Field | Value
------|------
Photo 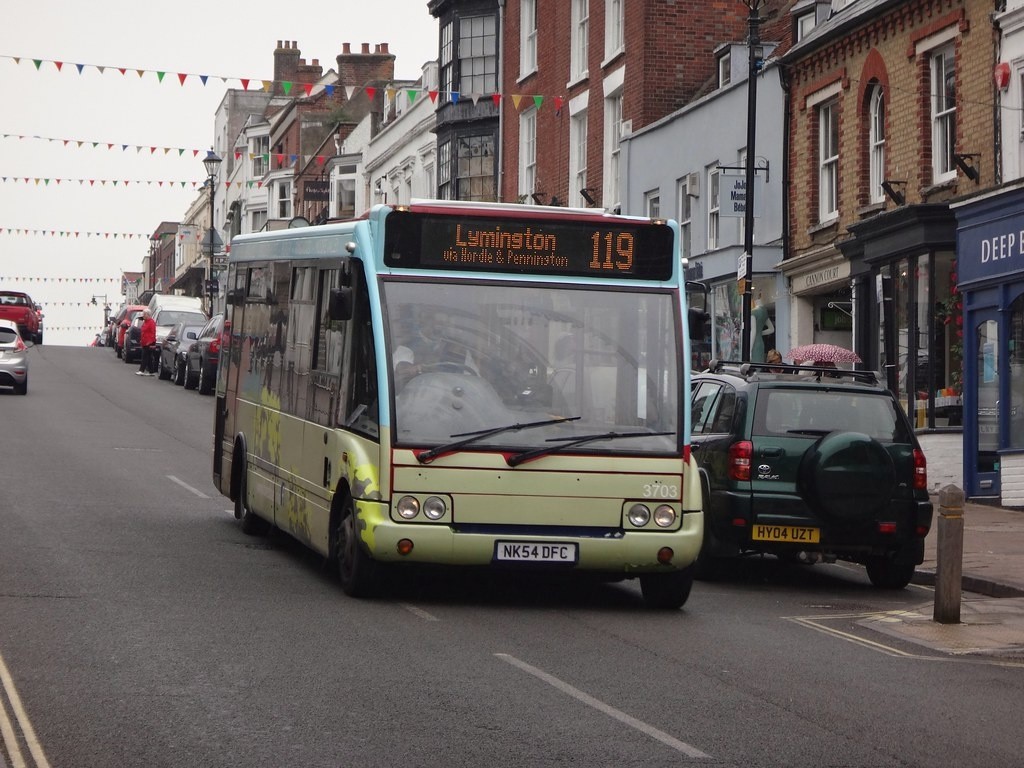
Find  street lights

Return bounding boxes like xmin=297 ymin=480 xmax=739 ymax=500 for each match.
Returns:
xmin=202 ymin=149 xmax=223 ymax=322
xmin=90 ymin=293 xmax=107 ymax=327
xmin=149 ymin=231 xmax=162 ymax=295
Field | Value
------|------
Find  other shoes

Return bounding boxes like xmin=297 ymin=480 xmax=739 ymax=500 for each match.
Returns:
xmin=135 ymin=371 xmax=144 ymax=376
xmin=145 ymin=372 xmax=155 ymax=376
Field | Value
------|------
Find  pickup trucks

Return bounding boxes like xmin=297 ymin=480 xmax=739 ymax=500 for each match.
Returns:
xmin=0 ymin=290 xmax=45 ymax=345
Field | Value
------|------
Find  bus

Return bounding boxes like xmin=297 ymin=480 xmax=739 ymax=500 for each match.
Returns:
xmin=207 ymin=197 xmax=717 ymax=613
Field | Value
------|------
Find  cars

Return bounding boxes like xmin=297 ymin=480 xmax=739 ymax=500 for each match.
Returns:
xmin=98 ymin=292 xmax=231 ymax=395
xmin=0 ymin=317 xmax=35 ymax=395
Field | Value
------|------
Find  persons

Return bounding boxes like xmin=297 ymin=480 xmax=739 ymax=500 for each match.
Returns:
xmin=748 ymin=299 xmax=775 ymax=363
xmin=135 ymin=308 xmax=157 ymax=377
xmin=763 ymin=348 xmax=791 ymax=373
xmin=392 ymin=298 xmax=481 ymax=383
xmin=811 ymin=360 xmax=841 ymax=378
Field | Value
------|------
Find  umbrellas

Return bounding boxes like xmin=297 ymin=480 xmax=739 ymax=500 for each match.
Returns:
xmin=786 ymin=344 xmax=863 ymax=363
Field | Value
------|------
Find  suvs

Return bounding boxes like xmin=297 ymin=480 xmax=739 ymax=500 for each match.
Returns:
xmin=683 ymin=357 xmax=935 ymax=592
